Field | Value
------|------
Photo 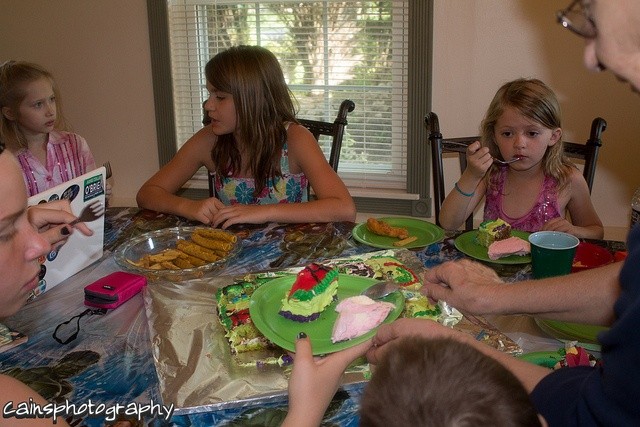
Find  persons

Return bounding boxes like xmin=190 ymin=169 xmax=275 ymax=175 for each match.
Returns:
xmin=0 ymin=138 xmax=373 ymax=427
xmin=366 ymin=0 xmax=637 ymax=427
xmin=137 ymin=44 xmax=356 ymax=230
xmin=437 ymin=77 xmax=604 ymax=241
xmin=357 ymin=333 xmax=550 ymax=427
xmin=0 ymin=61 xmax=113 ymax=200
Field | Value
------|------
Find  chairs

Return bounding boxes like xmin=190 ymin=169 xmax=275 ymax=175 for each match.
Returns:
xmin=421 ymin=110 xmax=608 ymax=234
xmin=199 ymin=98 xmax=356 ymax=202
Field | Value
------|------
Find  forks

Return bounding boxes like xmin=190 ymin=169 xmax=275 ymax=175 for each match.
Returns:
xmin=441 ymin=141 xmax=520 ymax=166
xmin=102 ymin=161 xmax=113 ymax=180
xmin=69 ymin=200 xmax=107 ymax=227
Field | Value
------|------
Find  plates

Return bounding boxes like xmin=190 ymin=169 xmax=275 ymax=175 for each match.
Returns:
xmin=249 ymin=273 xmax=405 ymax=356
xmin=571 ymin=242 xmax=614 ymax=273
xmin=351 ymin=217 xmax=443 ymax=249
xmin=113 ymin=224 xmax=243 ymax=281
xmin=454 ymin=230 xmax=533 ymax=264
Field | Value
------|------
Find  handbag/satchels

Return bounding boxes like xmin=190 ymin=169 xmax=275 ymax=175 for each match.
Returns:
xmin=51 ymin=270 xmax=148 ymax=345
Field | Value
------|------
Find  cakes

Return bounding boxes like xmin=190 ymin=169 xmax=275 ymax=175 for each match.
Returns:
xmin=549 ymin=340 xmax=597 ymax=374
xmin=216 ymin=254 xmax=443 ymax=381
xmin=476 ymin=218 xmax=512 ymax=248
xmin=279 ymin=262 xmax=339 ymax=320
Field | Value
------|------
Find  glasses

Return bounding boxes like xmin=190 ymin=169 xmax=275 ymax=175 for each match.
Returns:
xmin=557 ymin=2 xmax=599 ymax=39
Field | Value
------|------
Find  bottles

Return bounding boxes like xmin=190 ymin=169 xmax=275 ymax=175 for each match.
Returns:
xmin=630 ymin=188 xmax=640 ymax=233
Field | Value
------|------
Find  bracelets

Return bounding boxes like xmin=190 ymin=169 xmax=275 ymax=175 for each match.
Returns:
xmin=453 ymin=182 xmax=474 ymax=197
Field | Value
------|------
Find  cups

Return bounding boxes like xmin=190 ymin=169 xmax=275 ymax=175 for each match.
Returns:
xmin=528 ymin=230 xmax=580 ymax=279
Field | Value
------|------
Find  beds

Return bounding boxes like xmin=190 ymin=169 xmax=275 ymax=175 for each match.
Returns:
xmin=0 ymin=207 xmax=640 ymax=426
